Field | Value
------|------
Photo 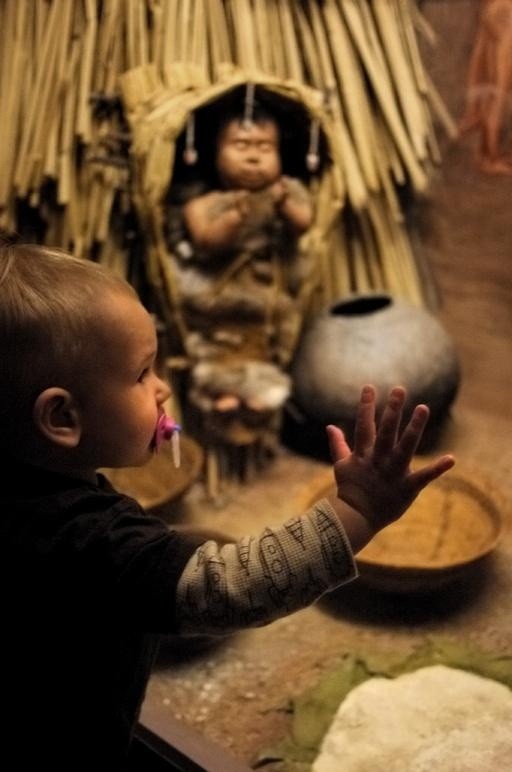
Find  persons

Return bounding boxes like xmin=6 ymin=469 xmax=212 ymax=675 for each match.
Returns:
xmin=179 ymin=98 xmax=316 ymax=415
xmin=0 ymin=248 xmax=454 ymax=772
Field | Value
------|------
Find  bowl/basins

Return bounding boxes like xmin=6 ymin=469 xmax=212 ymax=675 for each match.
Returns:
xmin=305 ymin=465 xmax=507 ymax=594
xmin=95 ymin=435 xmax=204 ymax=523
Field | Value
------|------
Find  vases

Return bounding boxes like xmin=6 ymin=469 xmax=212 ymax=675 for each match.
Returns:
xmin=285 ymin=290 xmax=461 ymax=458
